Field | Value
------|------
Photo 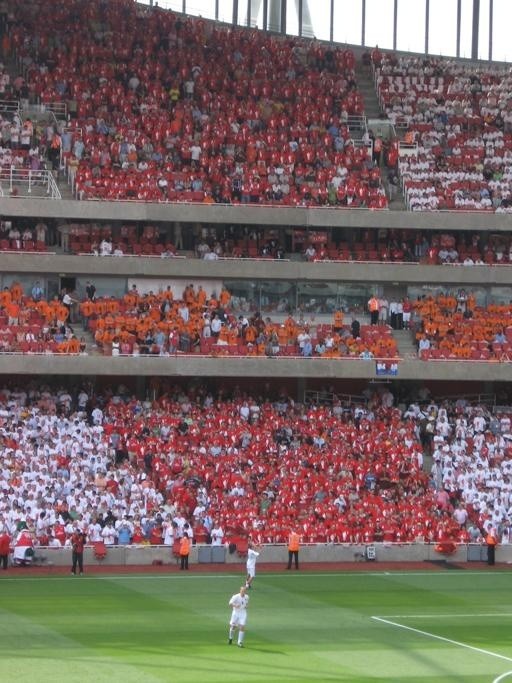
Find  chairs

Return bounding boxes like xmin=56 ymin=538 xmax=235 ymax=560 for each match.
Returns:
xmin=0 ymin=0 xmax=511 ymax=362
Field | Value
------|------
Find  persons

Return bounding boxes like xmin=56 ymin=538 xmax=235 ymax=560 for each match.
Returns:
xmin=2 ymin=218 xmax=512 ymax=265
xmin=227 ymin=586 xmax=250 ymax=647
xmin=71 ymin=529 xmax=86 ymax=573
xmin=486 ymin=523 xmax=498 ymax=565
xmin=0 ymin=531 xmax=11 ymax=568
xmin=1 ymin=0 xmax=511 ymax=213
xmin=244 ymin=542 xmax=266 ymax=589
xmin=0 ymin=379 xmax=512 ymax=545
xmin=0 ymin=280 xmax=512 ymax=362
xmin=179 ymin=532 xmax=192 ymax=569
xmin=287 ymin=528 xmax=300 ymax=569
